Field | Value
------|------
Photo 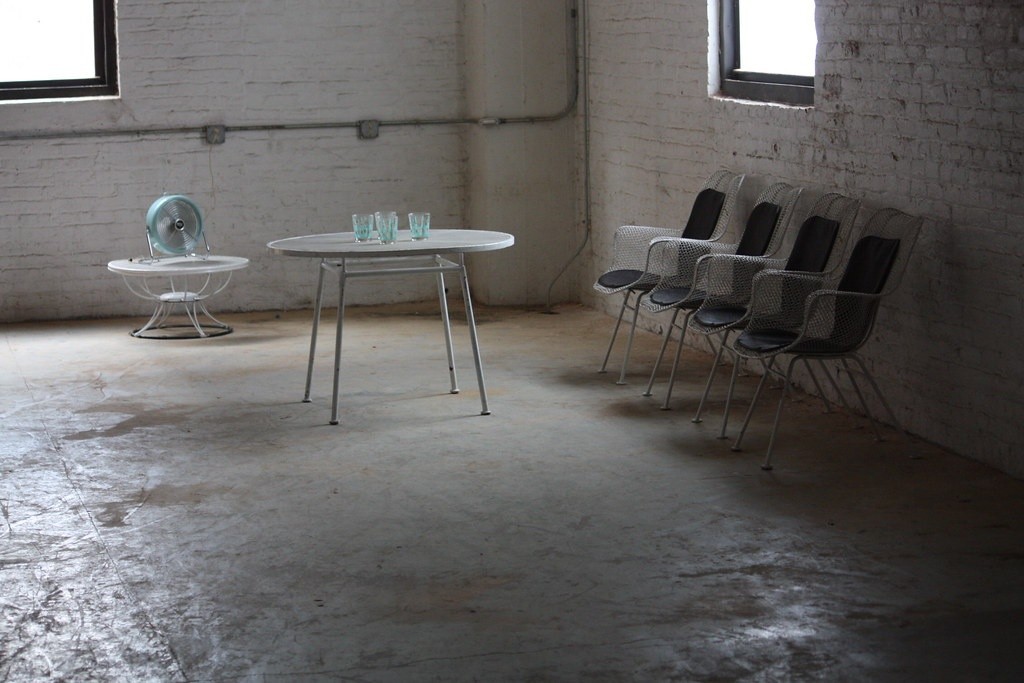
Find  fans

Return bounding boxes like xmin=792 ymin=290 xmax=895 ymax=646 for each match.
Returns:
xmin=138 ymin=194 xmax=211 ymax=266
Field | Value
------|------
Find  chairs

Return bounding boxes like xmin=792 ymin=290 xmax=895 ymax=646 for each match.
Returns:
xmin=593 ymin=171 xmax=927 ymax=469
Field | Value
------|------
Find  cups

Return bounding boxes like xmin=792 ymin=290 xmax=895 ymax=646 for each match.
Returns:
xmin=375 ymin=211 xmax=398 ymax=245
xmin=352 ymin=213 xmax=374 ymax=243
xmin=407 ymin=212 xmax=430 ymax=242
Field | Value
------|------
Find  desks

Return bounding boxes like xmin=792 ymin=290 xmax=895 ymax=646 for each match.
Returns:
xmin=107 ymin=253 xmax=249 ymax=339
xmin=267 ymin=227 xmax=514 ymax=425
